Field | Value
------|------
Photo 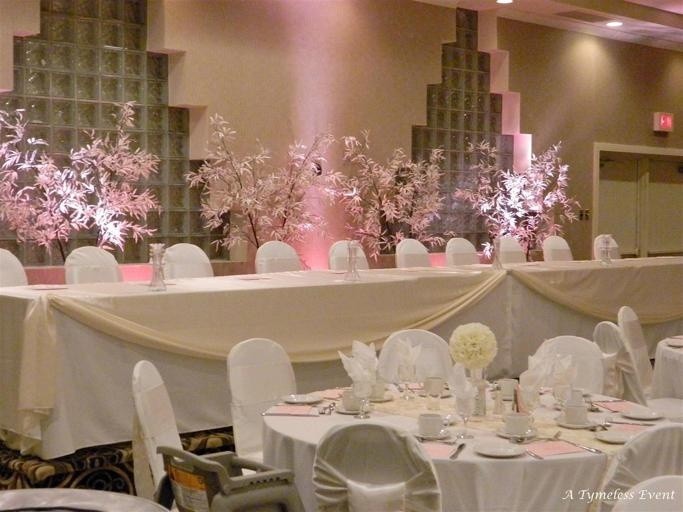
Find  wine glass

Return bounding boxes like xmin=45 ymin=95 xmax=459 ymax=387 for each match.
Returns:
xmin=455 ymin=396 xmax=473 ymax=439
xmin=352 ymin=380 xmax=371 ymax=420
xmin=394 ymin=358 xmax=417 ymax=401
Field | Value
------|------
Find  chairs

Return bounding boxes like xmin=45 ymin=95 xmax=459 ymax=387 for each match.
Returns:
xmin=528 ymin=333 xmax=605 ymax=396
xmin=160 ymin=242 xmax=215 ymax=278
xmin=131 ymin=359 xmax=260 ymax=508
xmin=445 ymin=237 xmax=479 ymax=263
xmin=225 ymin=337 xmax=300 ymax=477
xmin=498 ymin=237 xmax=526 ymax=263
xmin=541 ymin=236 xmax=574 ymax=261
xmin=255 ymin=240 xmax=303 ymax=274
xmin=154 ymin=441 xmax=306 ymax=510
xmin=311 ymin=417 xmax=443 ymax=511
xmin=591 ymin=420 xmax=682 ymax=512
xmin=63 ymin=245 xmax=125 ymax=283
xmin=328 ymin=240 xmax=368 ymax=269
xmin=0 ymin=248 xmax=29 ymax=286
xmin=593 ymin=234 xmax=622 ymax=258
xmin=614 ymin=472 xmax=680 ymax=510
xmin=395 ymin=238 xmax=431 ymax=268
xmin=378 ymin=327 xmax=452 ymax=380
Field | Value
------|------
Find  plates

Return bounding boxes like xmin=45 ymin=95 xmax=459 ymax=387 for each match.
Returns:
xmin=667 ymin=339 xmax=682 ymax=347
xmin=336 ymin=406 xmax=370 ymax=414
xmin=420 ymin=391 xmax=450 ymax=398
xmin=279 ymin=393 xmax=324 ymax=405
xmin=414 ymin=428 xmax=451 ymax=440
xmin=371 ymin=394 xmax=393 ymax=402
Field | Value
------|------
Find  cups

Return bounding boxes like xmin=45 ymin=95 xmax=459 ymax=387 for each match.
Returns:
xmin=417 ymin=412 xmax=440 ymax=435
xmin=341 ymin=390 xmax=361 ymax=411
xmin=418 ymin=376 xmax=444 ymax=395
xmin=370 ymin=380 xmax=383 ymax=400
xmin=473 ymin=376 xmax=666 ymax=456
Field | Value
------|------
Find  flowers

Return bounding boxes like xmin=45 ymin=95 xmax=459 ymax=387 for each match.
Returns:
xmin=0 ymin=89 xmax=164 ymax=259
xmin=186 ymin=108 xmax=329 ymax=253
xmin=316 ymin=120 xmax=443 ymax=261
xmin=453 ymin=130 xmax=579 ymax=257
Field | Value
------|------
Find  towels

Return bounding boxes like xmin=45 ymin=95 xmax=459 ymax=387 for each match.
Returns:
xmin=339 ymin=338 xmax=379 ymax=390
xmin=528 ymin=344 xmax=552 ymax=378
xmin=549 ymin=351 xmax=578 ymax=384
xmin=453 ymin=362 xmax=478 ymax=417
xmin=393 ymin=333 xmax=421 ymax=367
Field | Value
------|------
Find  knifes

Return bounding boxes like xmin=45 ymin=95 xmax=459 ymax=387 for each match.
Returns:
xmin=145 ymin=283 xmax=176 ymax=285
xmin=33 ymin=287 xmax=67 ymax=291
xmin=393 ymin=382 xmax=403 ymax=392
xmin=240 ymin=277 xmax=268 ymax=281
xmin=322 ymin=396 xmax=339 ymax=401
xmin=449 ymin=444 xmax=467 ymax=465
xmin=261 ymin=411 xmax=320 ymax=417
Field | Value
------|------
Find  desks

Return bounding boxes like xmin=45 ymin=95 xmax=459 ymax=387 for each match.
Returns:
xmin=1 ymin=247 xmax=508 ymax=460
xmin=502 ymin=253 xmax=681 ymax=383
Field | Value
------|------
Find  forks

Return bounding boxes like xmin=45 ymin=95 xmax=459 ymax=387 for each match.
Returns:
xmin=319 ymin=401 xmax=335 ymax=415
